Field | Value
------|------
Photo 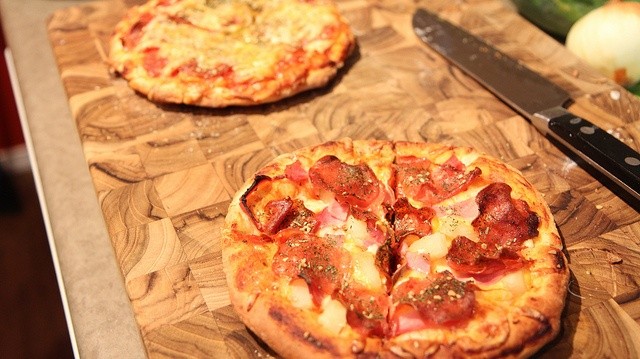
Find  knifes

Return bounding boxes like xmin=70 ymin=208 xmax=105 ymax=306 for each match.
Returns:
xmin=412 ymin=8 xmax=640 ymax=207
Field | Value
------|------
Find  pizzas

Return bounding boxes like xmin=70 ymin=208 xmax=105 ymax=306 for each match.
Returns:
xmin=220 ymin=137 xmax=571 ymax=359
xmin=107 ymin=0 xmax=356 ymax=108
